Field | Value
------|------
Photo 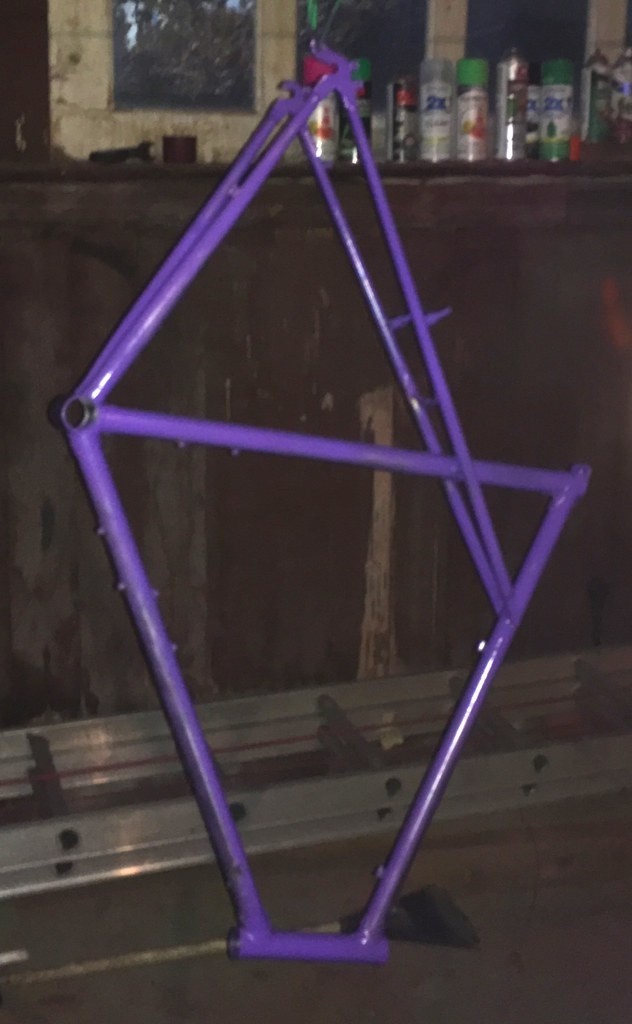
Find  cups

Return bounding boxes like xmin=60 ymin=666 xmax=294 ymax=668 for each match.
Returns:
xmin=162 ymin=136 xmax=196 ymax=163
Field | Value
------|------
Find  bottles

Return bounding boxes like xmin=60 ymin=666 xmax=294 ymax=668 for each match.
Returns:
xmin=304 ymin=45 xmax=632 ymax=173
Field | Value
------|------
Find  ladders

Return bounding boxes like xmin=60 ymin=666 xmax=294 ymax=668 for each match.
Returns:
xmin=0 ymin=641 xmax=630 ymax=903
